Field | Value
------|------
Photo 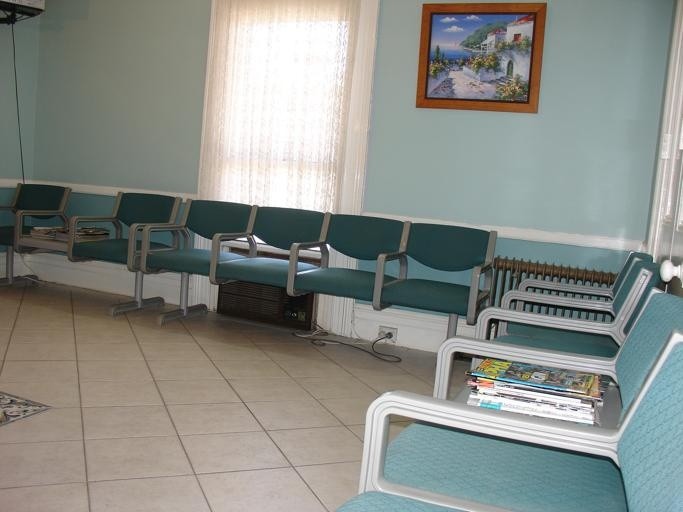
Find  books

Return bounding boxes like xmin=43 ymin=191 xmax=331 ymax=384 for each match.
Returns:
xmin=463 ymin=357 xmax=604 ymax=426
xmin=28 ymin=224 xmax=110 ymax=243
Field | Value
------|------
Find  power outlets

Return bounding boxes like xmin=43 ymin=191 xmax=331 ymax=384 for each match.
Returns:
xmin=377 ymin=325 xmax=398 ymax=344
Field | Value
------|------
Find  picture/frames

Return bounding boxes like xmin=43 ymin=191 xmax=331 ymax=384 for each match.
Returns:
xmin=414 ymin=2 xmax=548 ymax=114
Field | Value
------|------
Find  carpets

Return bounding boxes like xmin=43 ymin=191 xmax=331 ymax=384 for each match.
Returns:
xmin=0 ymin=389 xmax=53 ymax=430
